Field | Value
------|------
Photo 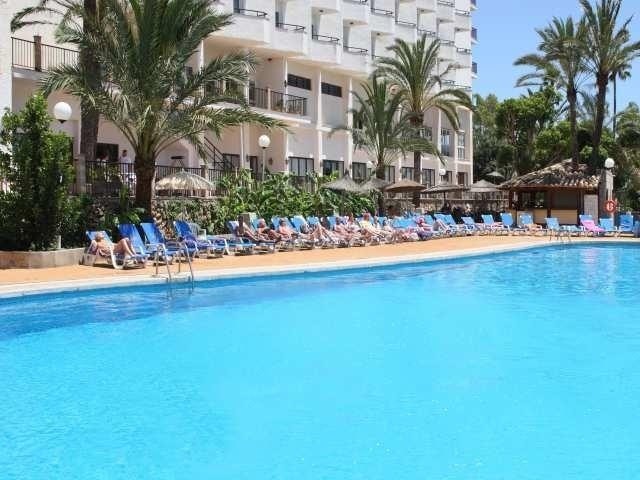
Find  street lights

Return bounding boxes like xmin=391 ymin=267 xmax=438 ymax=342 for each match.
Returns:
xmin=54 ymin=102 xmax=72 ymax=131
xmin=258 ymin=135 xmax=270 ymax=192
xmin=604 ymin=158 xmax=614 ymax=209
xmin=439 ymin=169 xmax=445 ymax=181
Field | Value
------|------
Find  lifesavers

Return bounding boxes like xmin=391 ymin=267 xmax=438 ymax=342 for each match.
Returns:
xmin=601 ymin=199 xmax=616 ymax=212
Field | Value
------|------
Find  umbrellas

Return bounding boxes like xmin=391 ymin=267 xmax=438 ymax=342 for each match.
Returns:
xmin=485 ymin=169 xmax=507 ymax=186
xmin=465 ymin=177 xmax=503 ymax=202
xmin=357 ymin=175 xmax=392 ymax=201
xmin=155 ymin=168 xmax=217 ymax=214
xmin=419 ymin=181 xmax=470 ymax=202
xmin=319 ymin=169 xmax=363 ymax=214
xmin=382 ymin=176 xmax=427 ymax=210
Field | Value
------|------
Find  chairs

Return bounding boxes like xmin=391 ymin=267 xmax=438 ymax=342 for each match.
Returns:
xmin=225 ymin=211 xmax=472 ymax=254
xmin=83 ymin=216 xmax=230 ymax=268
xmin=461 ymin=212 xmax=640 ymax=240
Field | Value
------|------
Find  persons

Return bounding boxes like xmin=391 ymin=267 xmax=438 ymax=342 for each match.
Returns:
xmin=118 ymin=149 xmax=132 ymax=182
xmin=94 ymin=232 xmax=135 ymax=259
xmin=278 ymin=218 xmax=315 ymax=242
xmin=333 ymin=201 xmax=452 ymax=247
xmin=95 ymin=150 xmax=109 ymax=175
xmin=256 ymin=218 xmax=285 ymax=243
xmin=235 ymin=215 xmax=264 ymax=244
xmin=300 ymin=221 xmax=335 ymax=243
